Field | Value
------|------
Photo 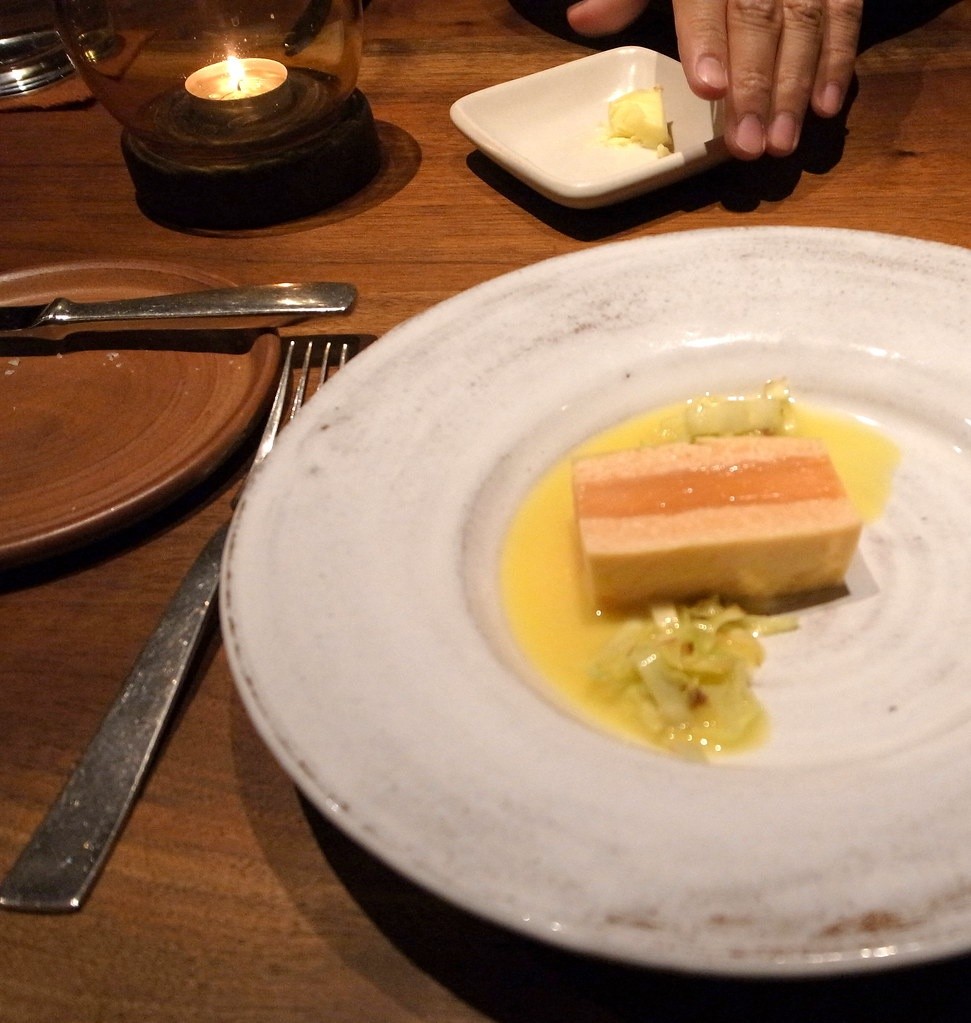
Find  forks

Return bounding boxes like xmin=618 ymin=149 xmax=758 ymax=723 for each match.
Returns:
xmin=0 ymin=341 xmax=348 ymax=913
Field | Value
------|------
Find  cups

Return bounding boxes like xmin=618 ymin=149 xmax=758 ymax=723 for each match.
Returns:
xmin=0 ymin=0 xmax=115 ymax=97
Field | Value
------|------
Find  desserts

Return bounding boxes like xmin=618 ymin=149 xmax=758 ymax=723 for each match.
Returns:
xmin=570 ymin=435 xmax=862 ymax=602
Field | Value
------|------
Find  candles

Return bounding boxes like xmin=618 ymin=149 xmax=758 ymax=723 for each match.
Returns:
xmin=184 ymin=58 xmax=288 ymax=124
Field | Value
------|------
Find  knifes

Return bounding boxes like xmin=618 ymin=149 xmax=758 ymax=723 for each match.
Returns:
xmin=0 ymin=283 xmax=358 ymax=330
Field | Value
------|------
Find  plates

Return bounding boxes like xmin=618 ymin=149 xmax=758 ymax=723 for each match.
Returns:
xmin=0 ymin=259 xmax=280 ymax=553
xmin=220 ymin=226 xmax=970 ymax=977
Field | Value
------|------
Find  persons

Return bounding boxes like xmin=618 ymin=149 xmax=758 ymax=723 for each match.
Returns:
xmin=566 ymin=0 xmax=864 ymax=159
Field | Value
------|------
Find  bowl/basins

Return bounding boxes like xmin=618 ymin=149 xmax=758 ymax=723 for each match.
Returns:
xmin=451 ymin=46 xmax=731 ymax=208
